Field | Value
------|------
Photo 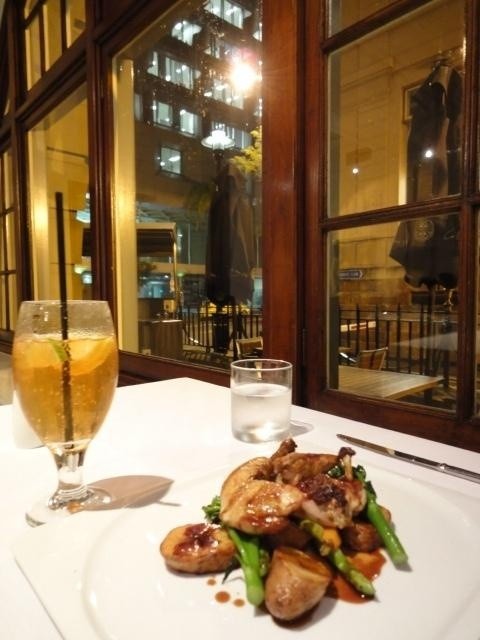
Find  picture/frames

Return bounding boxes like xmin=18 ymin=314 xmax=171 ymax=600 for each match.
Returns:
xmin=401 ymin=76 xmax=426 ymax=125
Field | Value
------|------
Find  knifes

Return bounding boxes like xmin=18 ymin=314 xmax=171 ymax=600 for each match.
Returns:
xmin=338 ymin=430 xmax=479 ymax=483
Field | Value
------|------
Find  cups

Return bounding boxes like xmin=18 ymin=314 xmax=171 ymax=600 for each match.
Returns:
xmin=228 ymin=359 xmax=294 ymax=441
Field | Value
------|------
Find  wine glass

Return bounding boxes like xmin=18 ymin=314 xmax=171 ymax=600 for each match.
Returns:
xmin=12 ymin=295 xmax=117 ymax=526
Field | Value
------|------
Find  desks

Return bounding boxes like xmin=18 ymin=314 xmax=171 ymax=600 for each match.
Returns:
xmin=337 ymin=364 xmax=446 ymax=405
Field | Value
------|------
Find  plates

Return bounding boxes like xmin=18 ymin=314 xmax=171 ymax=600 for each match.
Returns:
xmin=14 ymin=443 xmax=478 ymax=638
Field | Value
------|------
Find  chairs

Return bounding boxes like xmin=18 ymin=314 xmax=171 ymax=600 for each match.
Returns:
xmin=235 ymin=335 xmax=263 ymax=362
xmin=355 ymin=346 xmax=389 ymax=370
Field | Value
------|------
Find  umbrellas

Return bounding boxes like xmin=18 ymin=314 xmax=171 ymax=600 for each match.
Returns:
xmin=203 ymin=159 xmax=256 ymax=341
xmin=388 ymin=57 xmax=464 ymax=402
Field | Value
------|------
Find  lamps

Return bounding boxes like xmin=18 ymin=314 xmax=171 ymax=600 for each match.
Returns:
xmin=201 ymin=1 xmax=237 ymax=161
